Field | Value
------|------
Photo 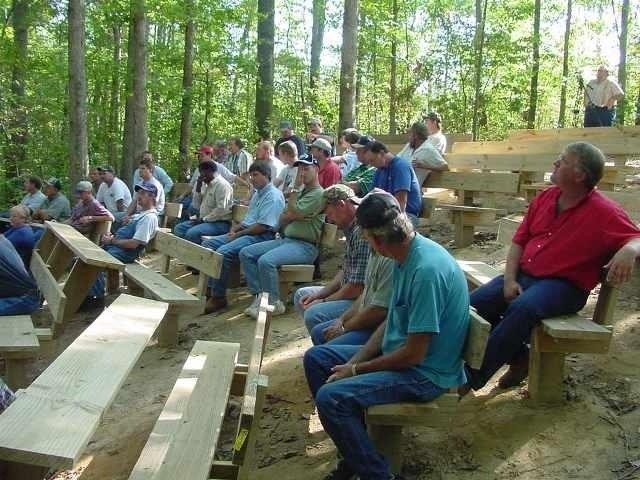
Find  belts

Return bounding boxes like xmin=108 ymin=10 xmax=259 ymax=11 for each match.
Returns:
xmin=286 ymin=236 xmax=316 ymax=247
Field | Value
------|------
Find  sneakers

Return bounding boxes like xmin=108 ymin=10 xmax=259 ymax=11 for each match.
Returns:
xmin=82 ymin=295 xmax=105 ymax=307
xmin=249 ymin=301 xmax=286 ymax=320
xmin=205 ymin=296 xmax=227 ymax=314
xmin=464 ymin=364 xmax=488 ymax=391
xmin=321 ymin=460 xmax=355 ymax=480
xmin=243 ymin=292 xmax=262 ymax=316
xmin=498 ymin=351 xmax=529 ymax=389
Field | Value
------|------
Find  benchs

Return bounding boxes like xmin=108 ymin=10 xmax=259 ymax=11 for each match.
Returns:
xmin=368 ymin=306 xmax=494 ymax=478
xmin=93 ymin=129 xmax=639 ymax=346
xmin=455 ymin=214 xmax=626 ymax=408
xmin=123 ymin=289 xmax=278 ymax=479
xmin=1 ymin=249 xmax=67 ymax=362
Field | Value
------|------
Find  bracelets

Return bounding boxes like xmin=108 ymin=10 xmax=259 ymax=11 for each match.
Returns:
xmin=351 ymin=363 xmax=358 ymax=376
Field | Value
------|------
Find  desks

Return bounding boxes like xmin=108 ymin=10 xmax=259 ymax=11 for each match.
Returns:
xmin=41 ymin=219 xmax=127 ymax=288
xmin=0 ymin=293 xmax=175 ymax=479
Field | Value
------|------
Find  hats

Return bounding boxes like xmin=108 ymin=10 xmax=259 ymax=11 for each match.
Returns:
xmin=421 ymin=112 xmax=441 ymax=123
xmin=96 ymin=164 xmax=114 ymax=172
xmin=406 ymin=123 xmax=428 ymax=141
xmin=355 ymin=192 xmax=402 ymax=228
xmin=193 ymin=145 xmax=214 ymax=154
xmin=322 ymin=184 xmax=365 ymax=206
xmin=135 ymin=183 xmax=157 ymax=194
xmin=43 ymin=178 xmax=61 ymax=189
xmin=293 ymin=135 xmax=376 ymax=167
xmin=72 ymin=181 xmax=93 ymax=193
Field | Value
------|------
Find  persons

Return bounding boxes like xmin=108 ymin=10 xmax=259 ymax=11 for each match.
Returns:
xmin=0 ymin=379 xmax=15 ymax=409
xmin=174 ymin=112 xmax=448 ymax=319
xmin=583 ymin=65 xmax=625 ymax=127
xmin=300 ymin=192 xmax=471 ymax=480
xmin=458 ymin=141 xmax=640 ymax=397
xmin=303 ymin=187 xmax=413 ymax=456
xmin=0 ymin=152 xmax=173 ymax=315
